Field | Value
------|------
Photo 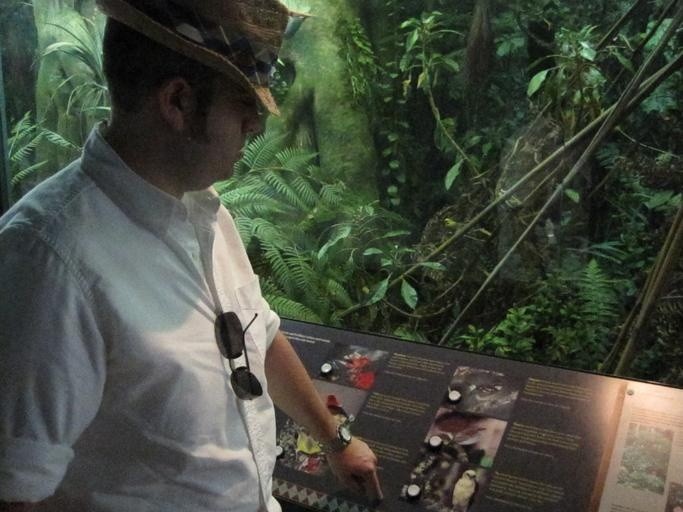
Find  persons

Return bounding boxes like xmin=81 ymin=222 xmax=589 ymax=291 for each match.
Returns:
xmin=0 ymin=0 xmax=384 ymax=512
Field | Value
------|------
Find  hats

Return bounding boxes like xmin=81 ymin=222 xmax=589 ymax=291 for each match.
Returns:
xmin=98 ymin=0 xmax=288 ymax=119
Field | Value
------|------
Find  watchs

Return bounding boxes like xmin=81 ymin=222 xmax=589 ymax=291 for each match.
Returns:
xmin=319 ymin=425 xmax=352 ymax=453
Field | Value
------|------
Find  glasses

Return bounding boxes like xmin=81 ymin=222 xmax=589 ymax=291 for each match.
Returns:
xmin=214 ymin=309 xmax=262 ymax=401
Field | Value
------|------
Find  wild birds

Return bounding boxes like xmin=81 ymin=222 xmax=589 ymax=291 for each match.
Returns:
xmin=451 ymin=470 xmax=476 ymax=512
